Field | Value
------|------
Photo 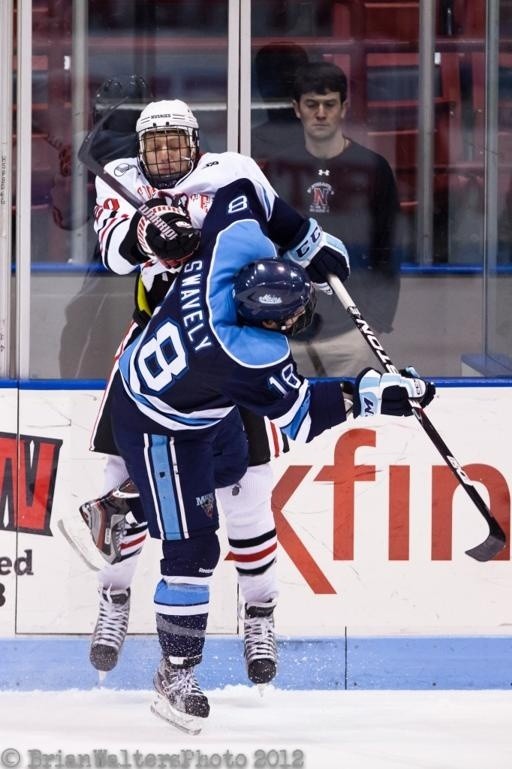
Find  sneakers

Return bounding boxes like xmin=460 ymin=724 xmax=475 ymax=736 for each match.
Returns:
xmin=244 ymin=599 xmax=277 ymax=683
xmin=90 ymin=584 xmax=130 ymax=671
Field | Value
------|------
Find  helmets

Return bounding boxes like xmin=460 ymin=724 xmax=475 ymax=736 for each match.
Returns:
xmin=136 ymin=99 xmax=199 ymax=189
xmin=231 ymin=258 xmax=318 ymax=336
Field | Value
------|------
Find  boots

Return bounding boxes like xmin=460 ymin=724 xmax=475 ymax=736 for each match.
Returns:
xmin=153 ymin=658 xmax=209 ymax=717
xmin=80 ymin=478 xmax=140 ymax=565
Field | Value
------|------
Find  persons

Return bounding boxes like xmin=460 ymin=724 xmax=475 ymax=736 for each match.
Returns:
xmin=87 ymin=95 xmax=285 ymax=682
xmin=74 ymin=175 xmax=436 ymax=720
xmin=259 ymin=58 xmax=401 ymax=382
xmin=47 ymin=73 xmax=159 ymax=380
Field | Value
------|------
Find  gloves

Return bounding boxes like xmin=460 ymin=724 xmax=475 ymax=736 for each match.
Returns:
xmin=131 ymin=198 xmax=200 ymax=269
xmin=278 ymin=218 xmax=351 ymax=296
xmin=354 ymin=366 xmax=435 ymax=418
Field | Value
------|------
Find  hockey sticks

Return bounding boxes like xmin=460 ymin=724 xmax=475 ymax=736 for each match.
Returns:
xmin=328 ymin=272 xmax=505 ymax=561
xmin=76 ymin=97 xmax=179 ymax=243
